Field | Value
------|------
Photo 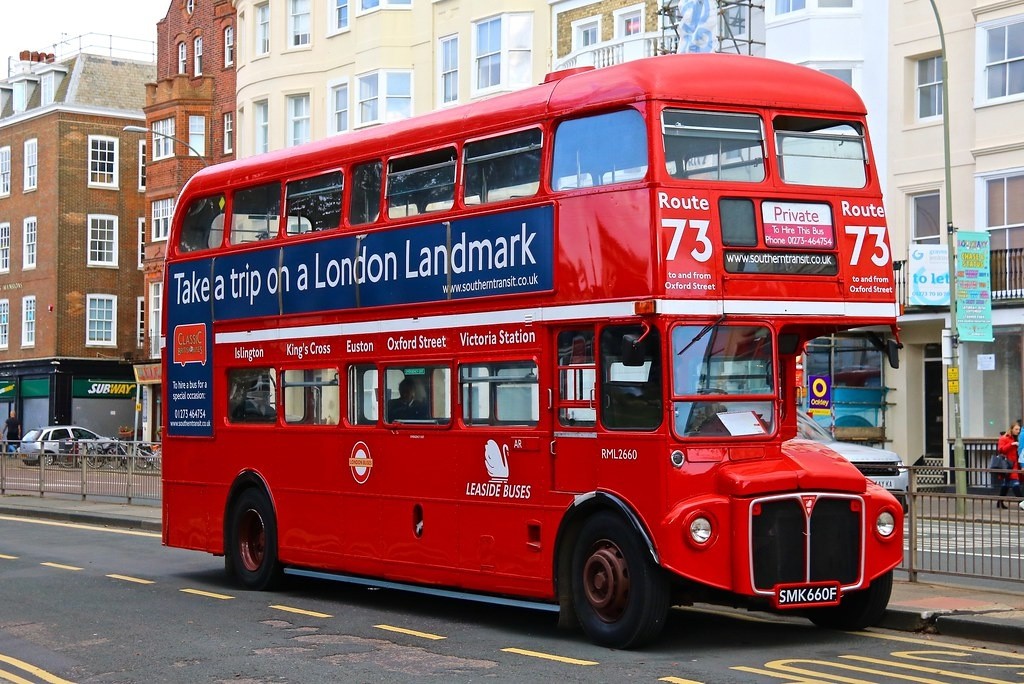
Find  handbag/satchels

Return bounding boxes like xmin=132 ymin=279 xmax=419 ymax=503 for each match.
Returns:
xmin=988 ymin=448 xmax=1014 ymax=479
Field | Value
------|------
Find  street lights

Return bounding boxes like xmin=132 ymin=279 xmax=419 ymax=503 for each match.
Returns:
xmin=123 ymin=125 xmax=208 ymax=170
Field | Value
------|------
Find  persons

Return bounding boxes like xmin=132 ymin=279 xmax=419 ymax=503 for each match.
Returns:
xmin=0 ymin=411 xmax=21 ymax=459
xmin=232 ymin=383 xmax=275 ymax=423
xmin=1018 ymin=427 xmax=1024 ymax=510
xmin=997 ymin=423 xmax=1021 ymax=509
xmin=387 ymin=379 xmax=430 ymax=424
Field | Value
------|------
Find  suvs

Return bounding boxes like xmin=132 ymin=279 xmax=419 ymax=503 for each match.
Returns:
xmin=795 ymin=406 xmax=909 ymax=518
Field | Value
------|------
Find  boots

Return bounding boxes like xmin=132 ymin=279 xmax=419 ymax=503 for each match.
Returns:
xmin=1013 ymin=486 xmax=1024 ymax=510
xmin=996 ymin=486 xmax=1008 ymax=509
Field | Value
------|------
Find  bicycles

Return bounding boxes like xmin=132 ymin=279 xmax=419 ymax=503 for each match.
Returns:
xmin=82 ymin=437 xmax=162 ymax=472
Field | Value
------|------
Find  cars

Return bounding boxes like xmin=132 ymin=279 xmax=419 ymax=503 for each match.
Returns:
xmin=19 ymin=425 xmax=111 ymax=466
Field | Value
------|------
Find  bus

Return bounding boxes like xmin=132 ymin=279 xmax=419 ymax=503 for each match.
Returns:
xmin=158 ymin=56 xmax=905 ymax=652
